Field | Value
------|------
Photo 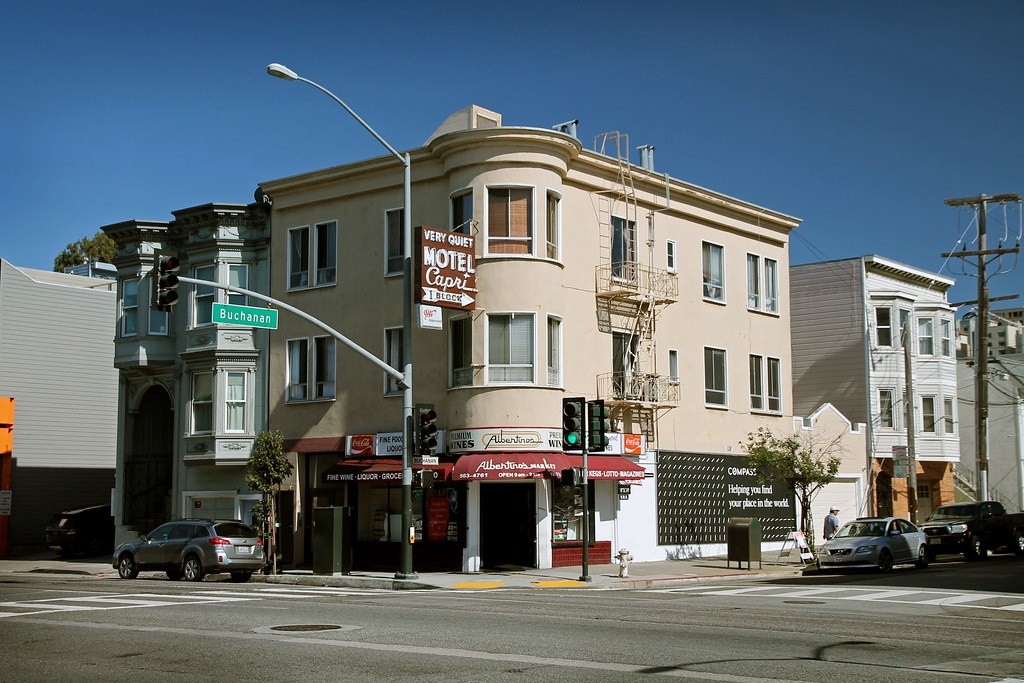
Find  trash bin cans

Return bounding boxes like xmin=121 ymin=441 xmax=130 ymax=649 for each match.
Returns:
xmin=727 ymin=517 xmax=762 ymax=571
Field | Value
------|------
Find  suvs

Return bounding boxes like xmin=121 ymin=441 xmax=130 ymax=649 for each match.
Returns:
xmin=919 ymin=501 xmax=1008 ymax=562
xmin=113 ymin=516 xmax=266 ymax=582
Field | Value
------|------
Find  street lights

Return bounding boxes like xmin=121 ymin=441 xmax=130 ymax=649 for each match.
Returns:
xmin=267 ymin=61 xmax=418 ymax=580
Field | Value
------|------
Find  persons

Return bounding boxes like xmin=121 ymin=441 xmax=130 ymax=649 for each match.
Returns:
xmin=824 ymin=506 xmax=840 ymax=541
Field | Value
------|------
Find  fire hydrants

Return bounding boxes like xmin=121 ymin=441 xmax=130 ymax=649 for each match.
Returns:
xmin=615 ymin=548 xmax=634 ymax=579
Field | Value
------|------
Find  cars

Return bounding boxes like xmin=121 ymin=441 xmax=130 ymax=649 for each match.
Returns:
xmin=44 ymin=505 xmax=116 ymax=557
xmin=998 ymin=512 xmax=1024 ymax=560
xmin=818 ymin=517 xmax=927 ymax=573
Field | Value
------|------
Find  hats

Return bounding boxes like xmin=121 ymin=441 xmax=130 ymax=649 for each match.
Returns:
xmin=830 ymin=506 xmax=840 ymax=510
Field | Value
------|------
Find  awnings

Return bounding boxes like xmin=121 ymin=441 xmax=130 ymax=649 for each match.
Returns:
xmin=565 ymin=456 xmax=645 ymax=479
xmin=451 ymin=452 xmax=576 ymax=480
xmin=321 ymin=456 xmax=454 ymax=486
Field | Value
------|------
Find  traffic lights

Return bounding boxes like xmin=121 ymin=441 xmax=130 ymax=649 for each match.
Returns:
xmin=563 ymin=397 xmax=585 ymax=451
xmin=588 ymin=399 xmax=609 ymax=452
xmin=416 ymin=408 xmax=438 ymax=455
xmin=156 ymin=254 xmax=180 ymax=312
